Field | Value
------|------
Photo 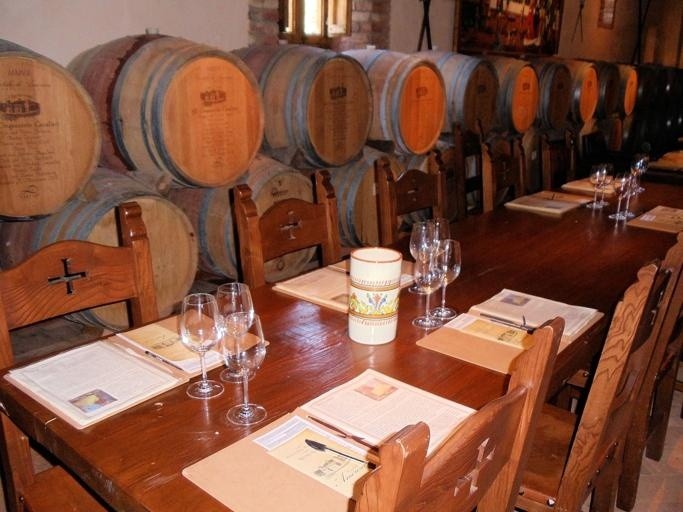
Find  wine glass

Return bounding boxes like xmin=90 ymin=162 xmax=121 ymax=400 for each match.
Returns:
xmin=588 ymin=153 xmax=648 ymax=228
xmin=217 ymin=282 xmax=255 ymax=382
xmin=180 ymin=293 xmax=225 ymax=400
xmin=409 ymin=217 xmax=461 ymax=331
xmin=223 ymin=311 xmax=266 ymax=424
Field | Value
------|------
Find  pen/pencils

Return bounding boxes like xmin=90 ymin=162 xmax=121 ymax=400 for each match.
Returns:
xmin=145 ymin=351 xmax=182 ymax=370
xmin=304 ymin=440 xmax=378 ymax=470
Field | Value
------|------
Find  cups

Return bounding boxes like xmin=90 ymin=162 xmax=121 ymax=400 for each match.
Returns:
xmin=348 ymin=247 xmax=403 ymax=345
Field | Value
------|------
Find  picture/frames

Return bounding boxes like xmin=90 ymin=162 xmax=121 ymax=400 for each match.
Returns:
xmin=598 ymin=2 xmax=620 ymax=32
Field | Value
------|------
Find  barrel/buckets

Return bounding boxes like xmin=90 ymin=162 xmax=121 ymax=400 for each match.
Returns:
xmin=585 ymin=59 xmax=622 ymax=113
xmin=0 ymin=39 xmax=103 ymax=221
xmin=636 ymin=62 xmax=683 ymax=118
xmin=614 ymin=62 xmax=641 ymax=114
xmin=526 ymin=58 xmax=574 ymax=131
xmin=66 ymin=33 xmax=267 ymax=191
xmin=391 ymin=139 xmax=470 ymax=228
xmin=228 ymin=43 xmax=373 ymax=170
xmin=256 ymin=143 xmax=408 ymax=247
xmin=556 ymin=58 xmax=602 ymax=125
xmin=340 ymin=44 xmax=447 ymax=157
xmin=1 ymin=165 xmax=199 ymax=334
xmin=461 ymin=117 xmax=683 ymax=222
xmin=476 ymin=55 xmax=541 ymax=134
xmin=98 ymin=151 xmax=318 ymax=283
xmin=409 ymin=49 xmax=499 ymax=134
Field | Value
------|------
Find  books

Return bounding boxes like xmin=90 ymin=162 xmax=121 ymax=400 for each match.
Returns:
xmin=630 ymin=202 xmax=682 ymax=233
xmin=562 ymin=169 xmax=636 ymax=197
xmin=650 ymin=146 xmax=681 ymax=171
xmin=416 ymin=288 xmax=605 ymax=378
xmin=10 ymin=307 xmax=264 ymax=433
xmin=273 ymin=246 xmax=435 ymax=318
xmin=505 ymin=189 xmax=595 ymax=219
xmin=183 ymin=369 xmax=480 ymax=512
